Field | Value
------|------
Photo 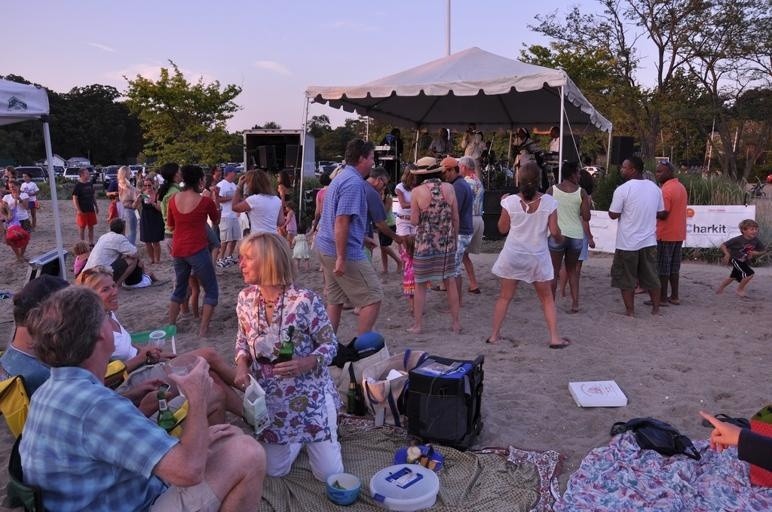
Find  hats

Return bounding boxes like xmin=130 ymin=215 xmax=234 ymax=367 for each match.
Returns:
xmin=224 ymin=166 xmax=240 ymax=173
xmin=409 ymin=156 xmax=444 ymax=175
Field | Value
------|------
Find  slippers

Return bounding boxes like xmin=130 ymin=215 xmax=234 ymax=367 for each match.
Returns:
xmin=549 ymin=337 xmax=571 ymax=349
xmin=702 ymin=413 xmax=751 ymax=430
xmin=486 ymin=337 xmax=494 ymax=344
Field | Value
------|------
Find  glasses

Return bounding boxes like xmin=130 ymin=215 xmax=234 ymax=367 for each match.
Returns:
xmin=253 ymin=333 xmax=280 ymax=367
xmin=80 ymin=269 xmax=93 ymax=285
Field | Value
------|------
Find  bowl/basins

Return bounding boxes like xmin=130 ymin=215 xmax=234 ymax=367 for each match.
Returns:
xmin=370 ymin=464 xmax=439 ymax=512
xmin=325 ymin=472 xmax=362 ymax=505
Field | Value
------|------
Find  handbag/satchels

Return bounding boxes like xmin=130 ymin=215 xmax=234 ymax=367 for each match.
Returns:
xmin=327 ymin=331 xmax=392 ymax=413
xmin=361 ymin=349 xmax=430 ymax=428
xmin=396 ymin=354 xmax=485 ymax=453
xmin=610 ymin=416 xmax=701 ymax=462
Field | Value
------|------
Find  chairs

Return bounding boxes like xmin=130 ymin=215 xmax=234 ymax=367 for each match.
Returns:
xmin=6 ymin=427 xmax=40 ymax=512
xmin=0 ymin=359 xmax=189 ymax=443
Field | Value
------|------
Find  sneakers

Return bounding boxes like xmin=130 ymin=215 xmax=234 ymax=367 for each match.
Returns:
xmin=468 ymin=287 xmax=481 ymax=294
xmin=214 ymin=255 xmax=241 ymax=276
xmin=431 ymin=285 xmax=447 ymax=291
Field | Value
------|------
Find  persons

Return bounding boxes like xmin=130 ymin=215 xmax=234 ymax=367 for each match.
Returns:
xmin=699 ymin=411 xmax=770 ymax=474
xmin=715 ymin=218 xmax=767 ymax=297
xmin=642 ymin=156 xmax=688 ymax=308
xmin=609 ymin=156 xmax=665 ymax=317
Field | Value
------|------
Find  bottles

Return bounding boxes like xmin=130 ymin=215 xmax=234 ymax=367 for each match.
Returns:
xmin=278 ymin=325 xmax=295 ymax=362
xmin=156 ymin=390 xmax=178 ymax=430
xmin=149 ymin=330 xmax=167 ymax=355
xmin=346 ymin=373 xmax=365 ymax=416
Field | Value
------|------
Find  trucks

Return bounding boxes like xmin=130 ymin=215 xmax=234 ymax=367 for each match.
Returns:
xmin=243 ymin=128 xmax=316 ymax=190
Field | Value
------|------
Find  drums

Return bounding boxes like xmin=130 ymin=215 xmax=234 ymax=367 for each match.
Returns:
xmin=541 ymin=152 xmax=559 ymax=167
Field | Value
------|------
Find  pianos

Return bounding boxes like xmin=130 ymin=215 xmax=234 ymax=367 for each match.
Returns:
xmin=378 ymin=154 xmax=395 ymax=161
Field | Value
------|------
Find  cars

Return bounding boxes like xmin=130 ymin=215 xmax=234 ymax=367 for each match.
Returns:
xmin=581 ymin=165 xmax=604 ymax=178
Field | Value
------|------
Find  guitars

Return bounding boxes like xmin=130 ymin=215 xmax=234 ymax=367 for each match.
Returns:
xmin=513 ymin=139 xmax=540 ymax=158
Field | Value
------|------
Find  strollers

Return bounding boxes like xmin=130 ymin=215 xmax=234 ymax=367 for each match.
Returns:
xmin=749 ymin=176 xmax=767 ymax=199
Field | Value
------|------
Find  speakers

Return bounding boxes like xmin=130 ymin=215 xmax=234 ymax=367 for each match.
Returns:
xmin=285 ymin=145 xmax=302 ymax=168
xmin=562 ymin=135 xmax=579 ymax=163
xmin=259 ymin=145 xmax=276 ymax=168
xmin=612 ymin=136 xmax=634 ymax=165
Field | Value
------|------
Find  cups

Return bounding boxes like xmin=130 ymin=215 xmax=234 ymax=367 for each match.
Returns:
xmin=170 ymin=355 xmax=199 ymax=396
